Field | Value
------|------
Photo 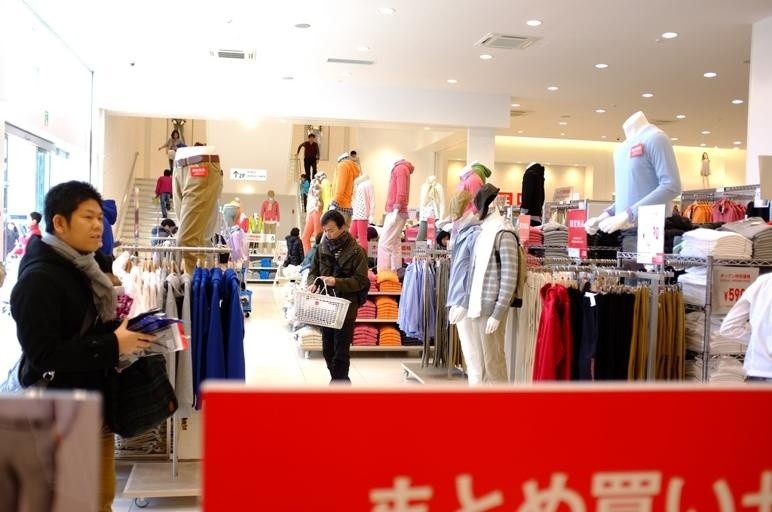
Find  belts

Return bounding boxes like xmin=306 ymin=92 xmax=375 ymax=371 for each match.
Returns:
xmin=173 ymin=154 xmax=220 ymax=168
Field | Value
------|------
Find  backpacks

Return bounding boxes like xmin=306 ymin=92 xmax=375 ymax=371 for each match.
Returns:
xmin=494 ymin=228 xmax=528 ymax=307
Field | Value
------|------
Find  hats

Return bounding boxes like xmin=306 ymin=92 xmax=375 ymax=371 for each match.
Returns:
xmin=450 ymin=191 xmax=471 ymax=221
xmin=475 ymin=183 xmax=500 ymax=220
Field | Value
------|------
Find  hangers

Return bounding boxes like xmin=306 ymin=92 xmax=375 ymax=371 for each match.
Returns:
xmin=554 ymin=202 xmax=575 ymax=211
xmin=120 ymin=250 xmax=236 ymax=274
xmin=525 ymin=255 xmax=675 ymax=295
xmin=691 ymin=192 xmax=740 ymax=204
xmin=407 ymin=247 xmax=452 ymax=262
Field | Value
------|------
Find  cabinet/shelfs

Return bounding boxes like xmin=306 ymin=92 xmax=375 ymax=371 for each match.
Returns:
xmin=347 ymin=291 xmax=442 ymax=352
xmin=247 ymin=255 xmax=282 ymax=283
xmin=666 ymin=252 xmax=772 ymax=383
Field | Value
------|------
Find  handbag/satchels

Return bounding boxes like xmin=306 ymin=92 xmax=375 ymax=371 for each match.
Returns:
xmin=103 ymin=354 xmax=178 ymax=438
xmin=1 ymin=361 xmax=48 ymax=394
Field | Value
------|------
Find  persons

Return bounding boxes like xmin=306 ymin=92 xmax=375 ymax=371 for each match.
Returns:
xmin=294 ymin=133 xmax=320 ymax=179
xmin=328 ymin=152 xmax=360 ymax=232
xmin=306 ymin=209 xmax=370 ymax=389
xmin=302 ymin=171 xmax=327 ymax=256
xmin=260 ymin=190 xmax=280 ymax=254
xmin=231 ymin=224 xmax=250 ymax=263
xmin=716 ymin=268 xmax=771 ymax=382
xmin=156 ymin=130 xmax=184 ymax=171
xmin=379 ymin=156 xmax=414 ymax=273
xmin=149 ymin=217 xmax=175 ymax=245
xmin=210 ymin=235 xmax=229 ymax=269
xmin=222 ymin=199 xmax=241 ymax=227
xmin=519 ymin=161 xmax=545 ymax=257
xmin=25 ymin=211 xmax=42 ymax=242
xmin=456 ymin=167 xmax=484 ymax=212
xmin=700 ymin=151 xmax=713 ymax=189
xmin=170 ymin=145 xmax=225 ymax=272
xmin=469 ymin=215 xmax=520 ymax=389
xmin=349 ymin=174 xmax=374 ymax=257
xmin=8 ymin=181 xmax=157 ymax=512
xmin=434 ymin=227 xmax=449 ymax=249
xmin=585 ymin=109 xmax=683 ymax=256
xmin=447 ymin=211 xmax=477 ymax=373
xmin=283 ymin=228 xmax=304 ymax=268
xmin=299 ymin=174 xmax=309 ymax=211
xmin=419 ymin=171 xmax=447 ymax=240
xmin=153 ymin=169 xmax=174 ymax=219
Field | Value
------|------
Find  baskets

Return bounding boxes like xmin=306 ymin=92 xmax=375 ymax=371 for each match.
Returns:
xmin=294 ymin=276 xmax=351 ymax=329
